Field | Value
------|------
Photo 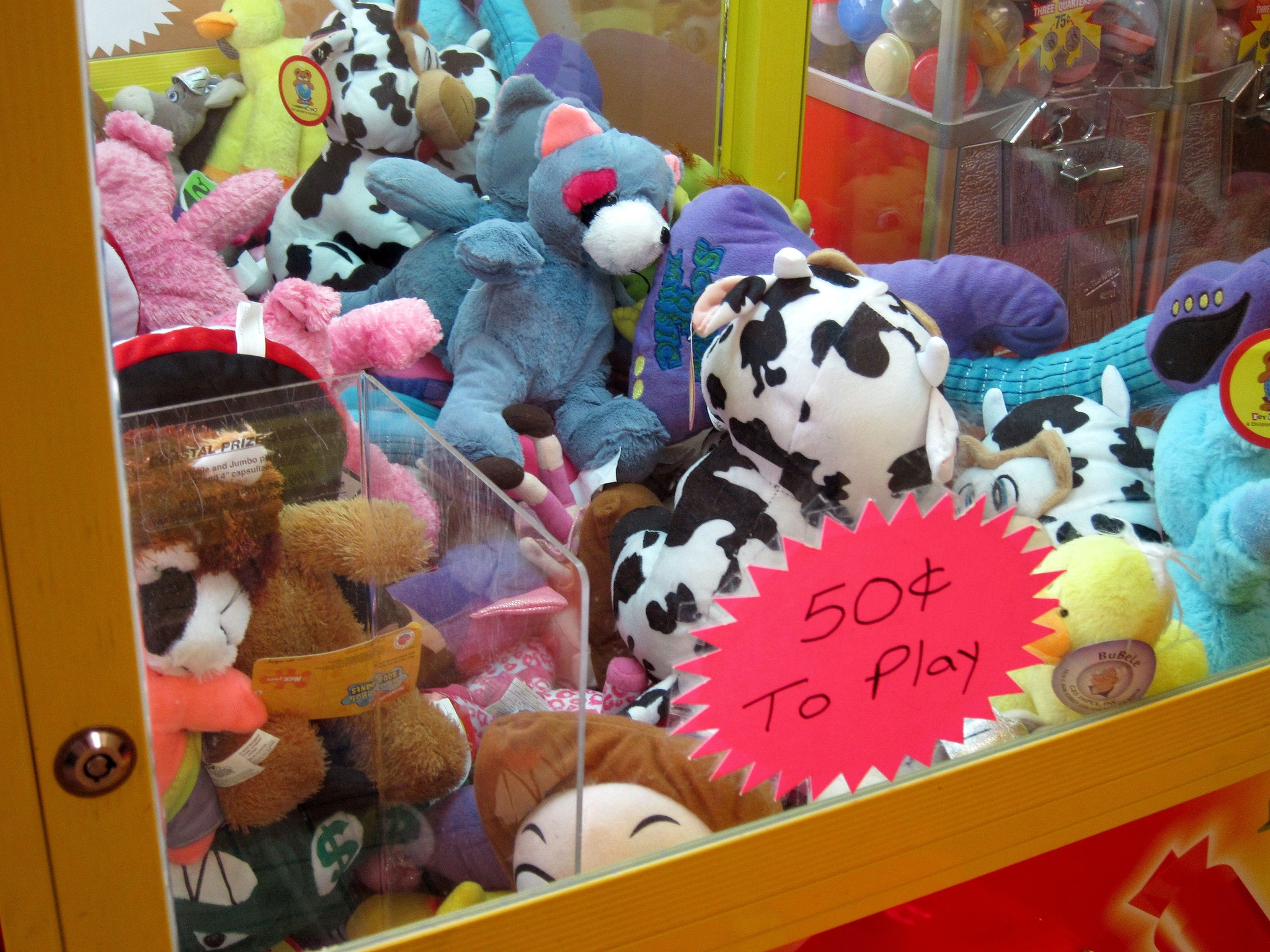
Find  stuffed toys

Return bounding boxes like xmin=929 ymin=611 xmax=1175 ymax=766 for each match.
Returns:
xmin=96 ymin=0 xmax=1270 ymax=952
